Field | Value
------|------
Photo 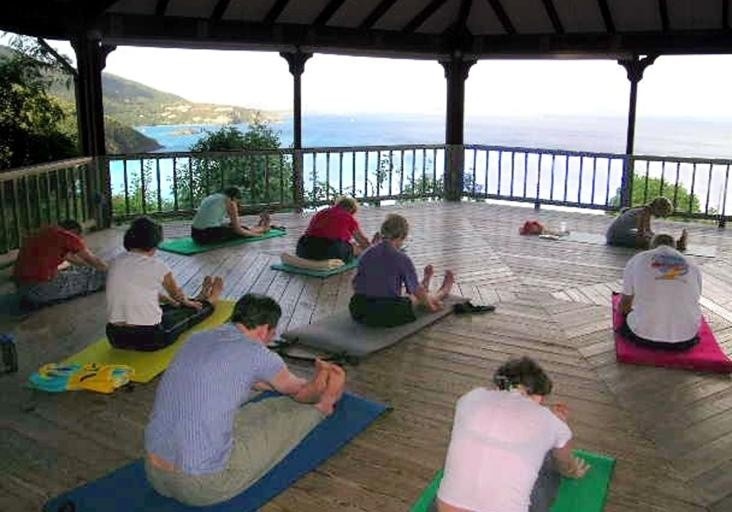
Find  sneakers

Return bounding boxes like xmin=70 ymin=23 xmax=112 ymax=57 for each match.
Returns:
xmin=1 ymin=304 xmax=29 ymax=321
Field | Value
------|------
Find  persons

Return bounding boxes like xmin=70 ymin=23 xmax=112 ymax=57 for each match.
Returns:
xmin=615 ymin=234 xmax=700 ymax=352
xmin=423 ymin=355 xmax=591 ymax=511
xmin=297 ymin=195 xmax=380 ymax=264
xmin=607 ymin=197 xmax=688 ymax=252
xmin=349 ymin=214 xmax=454 ymax=327
xmin=191 ymin=187 xmax=271 ymax=244
xmin=105 ymin=217 xmax=225 ymax=352
xmin=144 ymin=293 xmax=346 ymax=508
xmin=14 ymin=219 xmax=110 ymax=305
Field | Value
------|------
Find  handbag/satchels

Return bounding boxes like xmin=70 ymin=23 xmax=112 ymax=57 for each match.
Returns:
xmin=0 ymin=333 xmax=18 ymax=376
xmin=520 ymin=221 xmax=543 ymax=235
